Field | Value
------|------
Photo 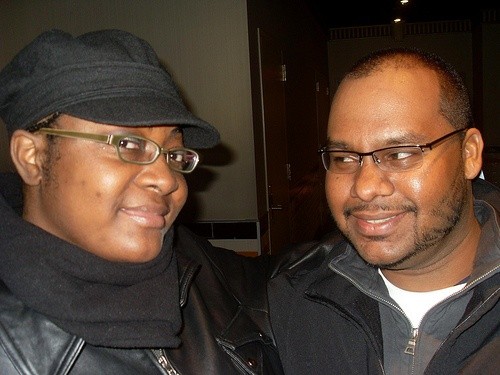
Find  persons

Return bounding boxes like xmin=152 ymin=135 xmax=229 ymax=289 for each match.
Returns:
xmin=262 ymin=47 xmax=500 ymax=375
xmin=0 ymin=29 xmax=347 ymax=375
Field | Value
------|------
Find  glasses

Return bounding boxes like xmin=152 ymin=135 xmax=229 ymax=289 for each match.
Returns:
xmin=319 ymin=127 xmax=471 ymax=175
xmin=33 ymin=126 xmax=200 ymax=173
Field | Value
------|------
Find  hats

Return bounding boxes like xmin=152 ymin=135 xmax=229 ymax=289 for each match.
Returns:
xmin=0 ymin=28 xmax=222 ymax=150
